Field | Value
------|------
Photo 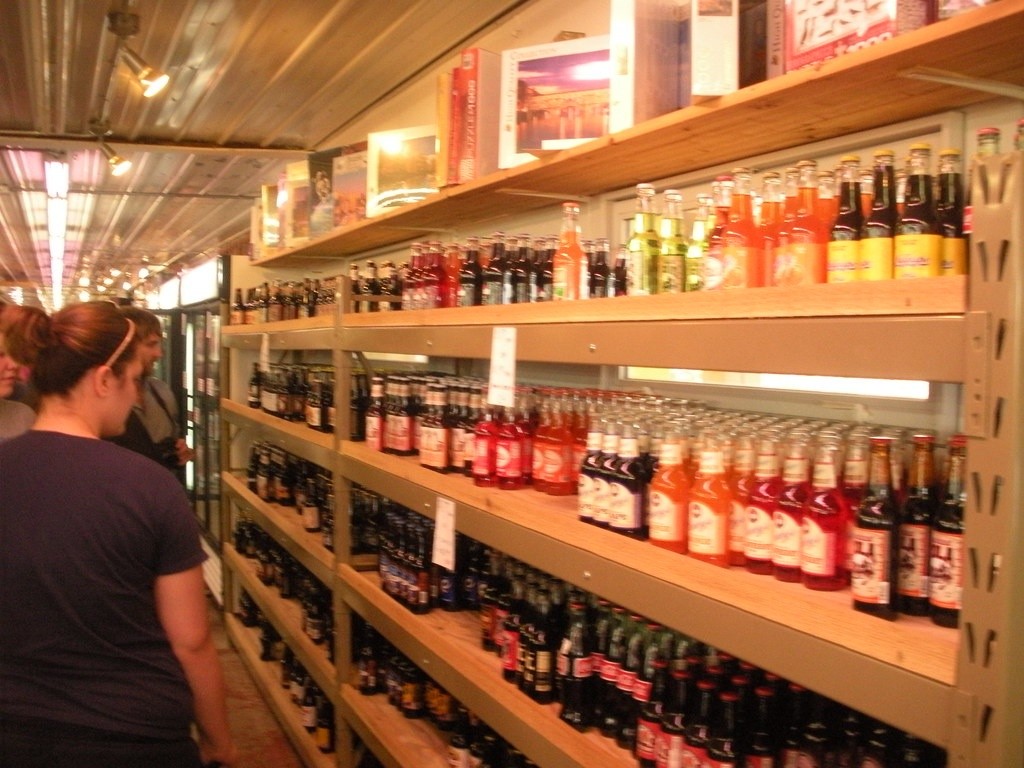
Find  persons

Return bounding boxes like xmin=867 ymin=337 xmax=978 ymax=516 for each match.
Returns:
xmin=100 ymin=307 xmax=193 ymax=490
xmin=0 ymin=301 xmax=238 ymax=768
xmin=0 ymin=290 xmax=37 ymax=442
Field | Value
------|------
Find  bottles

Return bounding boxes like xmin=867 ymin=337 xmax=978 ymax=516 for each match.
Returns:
xmin=249 ymin=362 xmax=335 ymax=434
xmin=358 ymin=622 xmax=537 ymax=768
xmin=581 ymin=238 xmax=627 ymax=298
xmin=962 ymin=118 xmax=1024 ymax=270
xmin=349 ymin=487 xmax=482 ymax=614
xmin=627 ymin=143 xmax=967 ymax=295
xmin=483 ymin=543 xmax=942 ymax=768
xmin=554 ymin=203 xmax=588 ymax=300
xmin=348 ymin=261 xmax=408 ymax=312
xmin=248 ymin=439 xmax=334 ymax=551
xmin=232 ymin=275 xmax=341 ymax=326
xmin=475 ymin=385 xmax=967 ymax=629
xmin=240 ymin=592 xmax=335 ymax=755
xmin=236 ymin=518 xmax=334 ymax=664
xmin=409 ymin=231 xmax=561 ymax=309
xmin=350 ymin=369 xmax=482 ymax=482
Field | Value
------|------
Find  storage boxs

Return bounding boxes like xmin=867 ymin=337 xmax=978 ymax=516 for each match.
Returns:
xmin=248 ymin=0 xmax=941 ymax=251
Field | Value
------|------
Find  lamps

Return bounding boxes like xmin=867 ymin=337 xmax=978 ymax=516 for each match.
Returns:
xmin=109 ymin=12 xmax=171 ymax=97
xmin=87 ymin=123 xmax=132 ymax=176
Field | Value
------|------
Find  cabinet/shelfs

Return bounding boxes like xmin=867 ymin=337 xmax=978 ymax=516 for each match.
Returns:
xmin=223 ymin=0 xmax=1024 ymax=768
